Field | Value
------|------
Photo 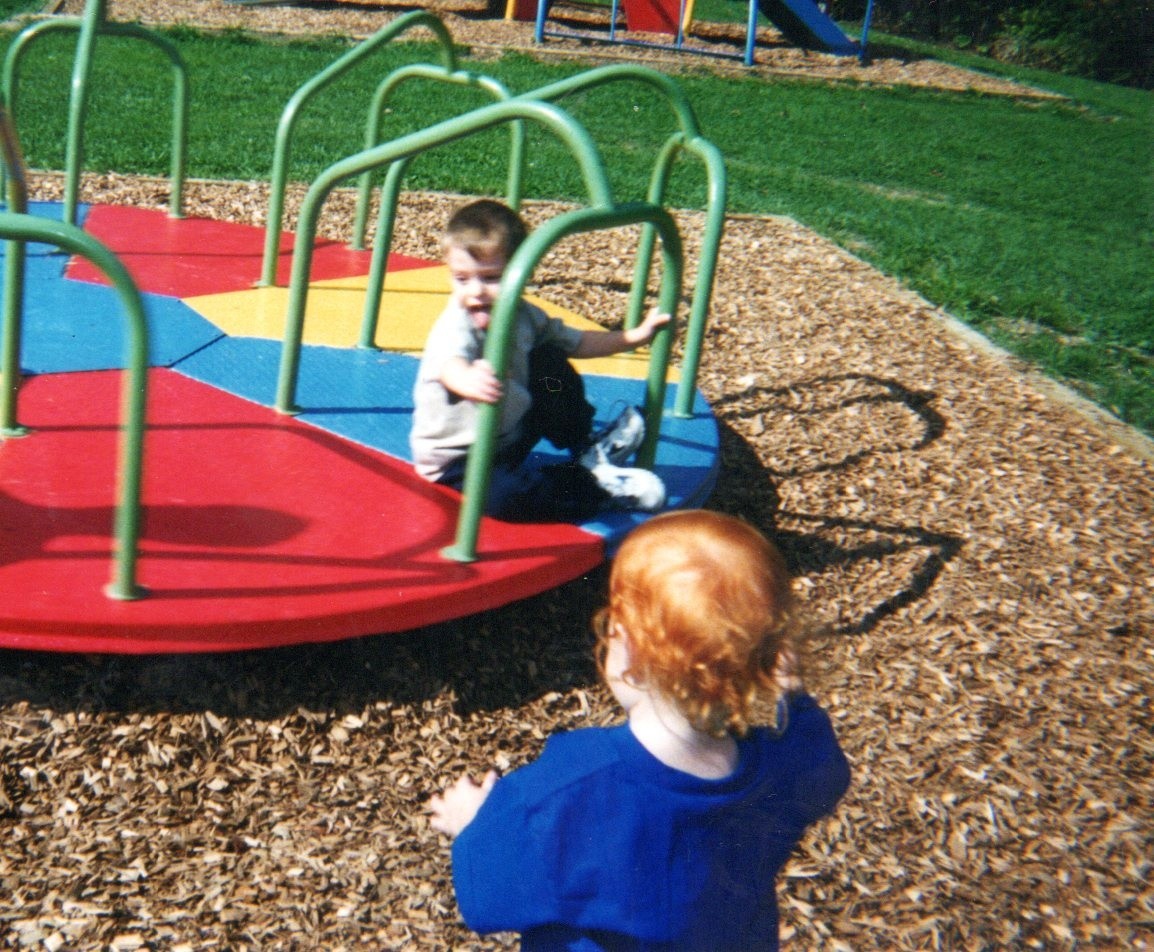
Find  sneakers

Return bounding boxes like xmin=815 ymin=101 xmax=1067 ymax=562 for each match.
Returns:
xmin=577 ymin=458 xmax=666 ymax=512
xmin=577 ymin=405 xmax=646 ymax=466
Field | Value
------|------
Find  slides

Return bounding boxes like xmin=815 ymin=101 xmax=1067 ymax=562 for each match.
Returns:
xmin=744 ymin=0 xmax=884 ymax=65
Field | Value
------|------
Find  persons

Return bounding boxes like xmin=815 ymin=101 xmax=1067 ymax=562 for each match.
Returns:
xmin=405 ymin=197 xmax=680 ymax=524
xmin=427 ymin=509 xmax=851 ymax=952
